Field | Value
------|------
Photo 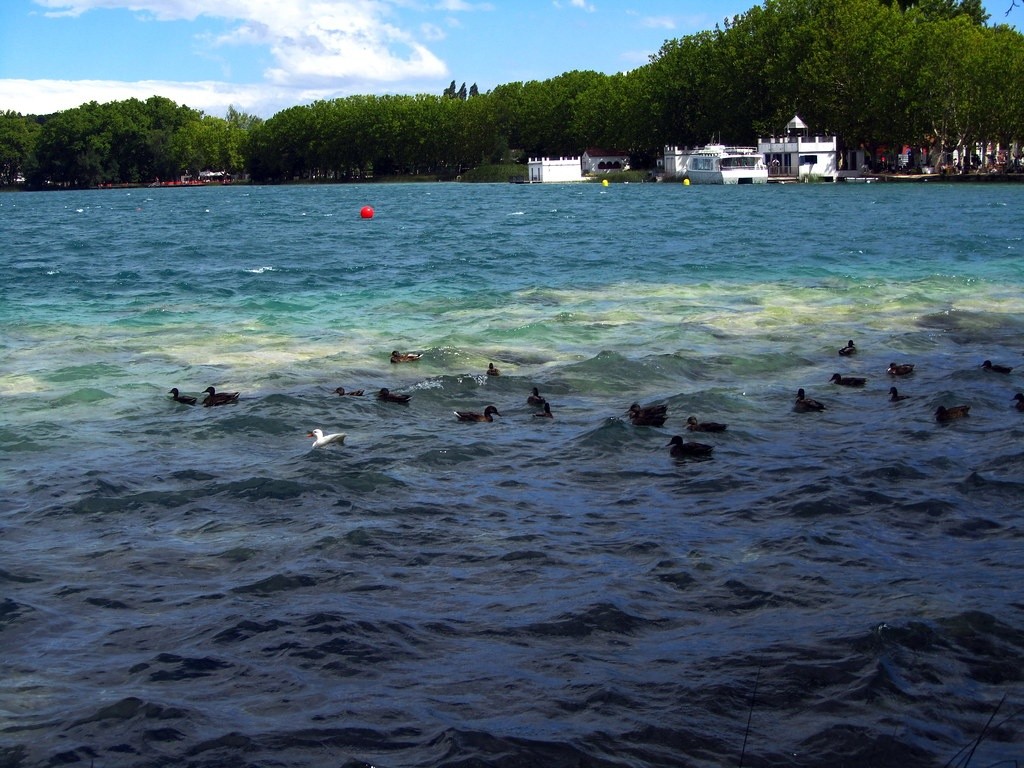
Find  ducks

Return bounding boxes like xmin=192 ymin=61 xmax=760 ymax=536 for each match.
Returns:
xmin=167 ymin=340 xmax=552 ymax=449
xmin=626 ymin=339 xmax=1023 ymax=453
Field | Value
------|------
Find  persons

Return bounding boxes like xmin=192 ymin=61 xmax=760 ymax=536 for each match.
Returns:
xmin=772 ymin=158 xmax=780 ymax=174
xmin=898 ymin=158 xmax=903 ymax=170
